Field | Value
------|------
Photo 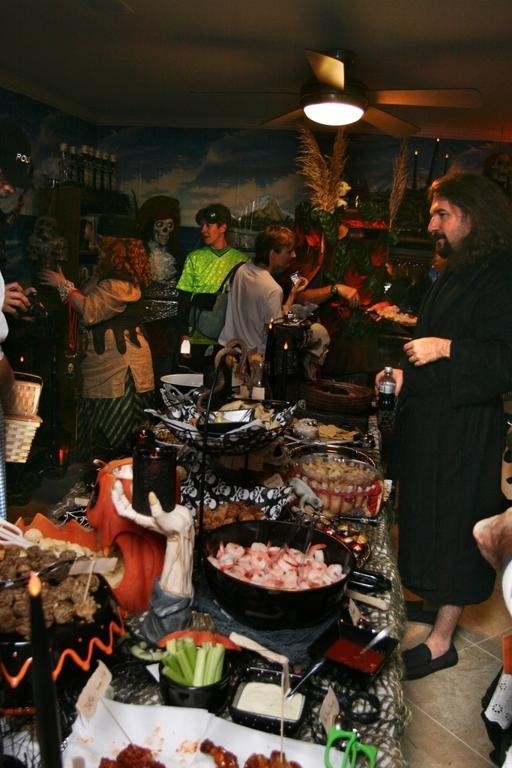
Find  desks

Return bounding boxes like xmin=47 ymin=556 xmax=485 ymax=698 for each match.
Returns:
xmin=48 ymin=415 xmax=415 ymax=767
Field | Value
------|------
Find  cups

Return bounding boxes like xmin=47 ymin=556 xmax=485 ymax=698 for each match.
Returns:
xmin=156 ymin=650 xmax=233 ymax=715
xmin=132 ymin=446 xmax=188 ymax=515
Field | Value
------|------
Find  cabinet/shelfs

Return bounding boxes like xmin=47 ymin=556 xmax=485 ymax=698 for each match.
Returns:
xmin=38 ymin=185 xmax=131 ymax=345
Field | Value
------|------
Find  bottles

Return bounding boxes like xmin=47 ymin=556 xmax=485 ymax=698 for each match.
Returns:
xmin=377 ymin=366 xmax=396 ymax=412
xmin=57 ymin=141 xmax=119 ymax=194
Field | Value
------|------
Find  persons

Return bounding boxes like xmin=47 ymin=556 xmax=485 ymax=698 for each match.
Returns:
xmin=2 ymin=182 xmax=155 ymax=505
xmin=375 ymin=171 xmax=512 ymax=680
xmin=1 ymin=121 xmax=40 ymax=320
xmin=137 ymin=198 xmax=360 ymax=397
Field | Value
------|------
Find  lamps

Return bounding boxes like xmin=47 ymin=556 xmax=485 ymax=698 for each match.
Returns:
xmin=298 ymin=96 xmax=366 ymax=129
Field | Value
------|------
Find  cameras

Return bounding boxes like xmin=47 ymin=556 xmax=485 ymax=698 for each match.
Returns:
xmin=289 ymin=270 xmax=304 ymax=286
xmin=11 ymin=288 xmax=48 ymax=320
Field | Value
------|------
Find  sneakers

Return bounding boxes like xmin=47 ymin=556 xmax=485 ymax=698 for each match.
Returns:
xmin=401 ymin=643 xmax=458 ymax=680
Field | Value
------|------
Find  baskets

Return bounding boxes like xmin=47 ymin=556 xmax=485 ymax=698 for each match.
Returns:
xmin=2 ymin=371 xmax=43 ymax=463
xmin=299 ymin=380 xmax=373 ymax=414
xmin=158 ymin=397 xmax=294 ymax=456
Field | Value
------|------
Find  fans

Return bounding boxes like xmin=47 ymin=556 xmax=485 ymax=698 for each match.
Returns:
xmin=179 ymin=41 xmax=487 ymax=138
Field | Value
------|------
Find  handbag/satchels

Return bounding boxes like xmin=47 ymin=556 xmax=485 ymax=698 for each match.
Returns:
xmin=185 ymin=261 xmax=245 ymax=339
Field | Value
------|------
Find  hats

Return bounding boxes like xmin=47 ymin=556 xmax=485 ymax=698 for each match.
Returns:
xmin=0 ymin=120 xmax=36 ymax=192
xmin=97 ymin=214 xmax=139 ymax=237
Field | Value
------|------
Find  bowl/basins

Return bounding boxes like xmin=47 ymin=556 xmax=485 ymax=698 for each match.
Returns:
xmin=204 ymin=520 xmax=354 ymax=631
xmin=229 ymin=666 xmax=310 ymax=736
xmin=161 ymin=371 xmax=245 ymax=392
xmin=304 ymin=620 xmax=401 ymax=689
